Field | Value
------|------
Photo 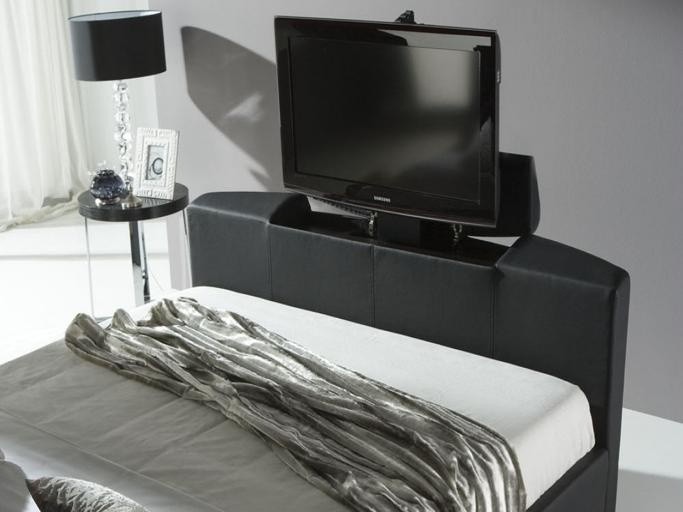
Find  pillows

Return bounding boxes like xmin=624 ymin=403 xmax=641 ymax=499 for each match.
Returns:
xmin=25 ymin=477 xmax=149 ymax=512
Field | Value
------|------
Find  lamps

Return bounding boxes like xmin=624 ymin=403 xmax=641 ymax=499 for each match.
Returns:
xmin=68 ymin=10 xmax=166 ymax=186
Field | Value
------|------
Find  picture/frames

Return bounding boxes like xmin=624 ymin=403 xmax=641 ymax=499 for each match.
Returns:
xmin=133 ymin=129 xmax=179 ymax=201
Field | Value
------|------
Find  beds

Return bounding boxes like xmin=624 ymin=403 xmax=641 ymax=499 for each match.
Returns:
xmin=1 ymin=191 xmax=630 ymax=512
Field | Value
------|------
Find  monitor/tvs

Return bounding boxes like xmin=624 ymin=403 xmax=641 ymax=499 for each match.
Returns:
xmin=274 ymin=16 xmax=501 ymax=227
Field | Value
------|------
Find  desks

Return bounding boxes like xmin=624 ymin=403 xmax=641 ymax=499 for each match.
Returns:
xmin=77 ymin=180 xmax=189 ymax=307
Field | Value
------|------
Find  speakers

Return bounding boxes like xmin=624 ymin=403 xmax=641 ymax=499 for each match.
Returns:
xmin=465 ymin=152 xmax=542 ymax=238
